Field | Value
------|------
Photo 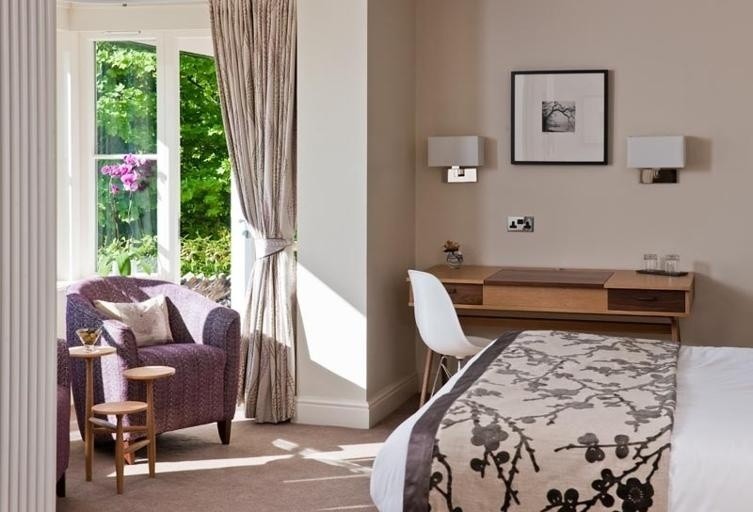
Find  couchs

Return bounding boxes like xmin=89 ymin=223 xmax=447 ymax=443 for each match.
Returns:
xmin=67 ymin=275 xmax=240 ymax=465
xmin=56 ymin=339 xmax=72 ymax=498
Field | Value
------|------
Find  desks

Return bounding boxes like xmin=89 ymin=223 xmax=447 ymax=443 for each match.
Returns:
xmin=68 ymin=346 xmax=176 ymax=495
xmin=406 ymin=264 xmax=695 ymax=410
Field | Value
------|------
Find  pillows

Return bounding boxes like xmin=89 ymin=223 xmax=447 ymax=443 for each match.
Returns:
xmin=93 ymin=294 xmax=174 ymax=347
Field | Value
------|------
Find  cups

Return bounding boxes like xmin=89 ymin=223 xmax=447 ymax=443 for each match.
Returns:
xmin=644 ymin=254 xmax=658 ymax=273
xmin=76 ymin=327 xmax=102 ymax=352
xmin=664 ymin=254 xmax=679 ymax=272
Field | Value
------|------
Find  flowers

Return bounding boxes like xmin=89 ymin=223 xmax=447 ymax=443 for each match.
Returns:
xmin=101 ymin=153 xmax=151 ymax=276
xmin=442 ymin=239 xmax=460 ymax=262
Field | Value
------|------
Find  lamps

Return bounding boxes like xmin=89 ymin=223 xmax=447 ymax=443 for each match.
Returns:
xmin=627 ymin=136 xmax=687 ymax=183
xmin=428 ymin=136 xmax=485 ymax=183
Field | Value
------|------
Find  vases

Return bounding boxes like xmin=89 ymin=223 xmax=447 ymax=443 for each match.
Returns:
xmin=446 ymin=253 xmax=463 ymax=269
xmin=112 ymin=261 xmax=136 ymax=275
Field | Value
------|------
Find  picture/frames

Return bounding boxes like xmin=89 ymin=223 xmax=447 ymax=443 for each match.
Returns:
xmin=510 ymin=69 xmax=608 ymax=165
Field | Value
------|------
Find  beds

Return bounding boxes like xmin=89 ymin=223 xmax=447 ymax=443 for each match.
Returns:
xmin=369 ymin=331 xmax=752 ymax=512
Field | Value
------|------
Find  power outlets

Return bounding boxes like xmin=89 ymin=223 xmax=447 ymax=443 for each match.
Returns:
xmin=503 ymin=213 xmax=538 ymax=238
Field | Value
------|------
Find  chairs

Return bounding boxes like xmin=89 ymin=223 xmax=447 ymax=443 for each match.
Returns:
xmin=408 ymin=269 xmax=493 ymax=409
